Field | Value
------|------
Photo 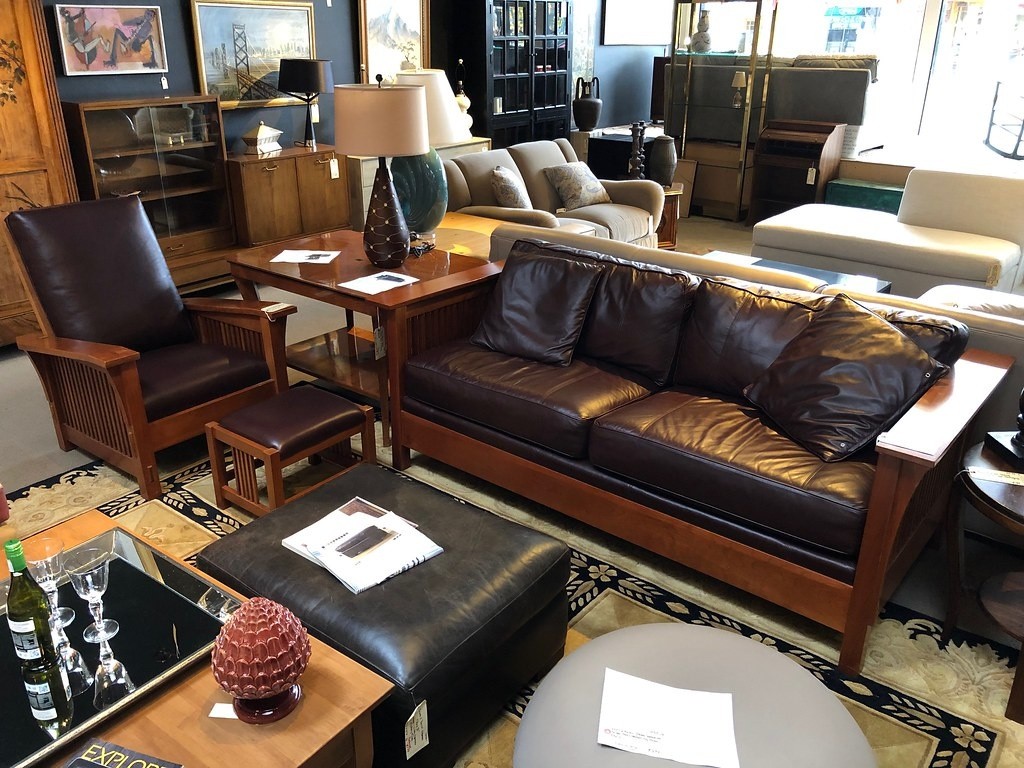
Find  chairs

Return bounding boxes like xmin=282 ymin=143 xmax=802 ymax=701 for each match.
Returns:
xmin=3 ymin=195 xmax=298 ymax=499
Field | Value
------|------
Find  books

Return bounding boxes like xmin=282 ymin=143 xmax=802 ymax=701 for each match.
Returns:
xmin=284 ymin=491 xmax=445 ymax=597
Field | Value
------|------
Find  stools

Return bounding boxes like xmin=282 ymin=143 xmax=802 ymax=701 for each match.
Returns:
xmin=206 ymin=380 xmax=376 ymax=518
xmin=195 ymin=458 xmax=572 ymax=768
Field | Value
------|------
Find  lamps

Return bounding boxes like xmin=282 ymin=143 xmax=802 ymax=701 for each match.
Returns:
xmin=277 ymin=58 xmax=335 ymax=147
xmin=731 ymin=71 xmax=746 ymax=107
xmin=334 ymin=74 xmax=431 ymax=269
xmin=391 ymin=70 xmax=472 ymax=238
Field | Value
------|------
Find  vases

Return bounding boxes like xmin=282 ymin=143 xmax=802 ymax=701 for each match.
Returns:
xmin=572 ymin=76 xmax=603 ymax=132
xmin=691 ymin=10 xmax=711 ymax=53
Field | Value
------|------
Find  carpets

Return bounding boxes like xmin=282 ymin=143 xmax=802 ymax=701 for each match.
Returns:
xmin=0 ymin=363 xmax=1024 ymax=768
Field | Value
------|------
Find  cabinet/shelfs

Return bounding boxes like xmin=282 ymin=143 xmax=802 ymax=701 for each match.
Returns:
xmin=58 ymin=0 xmax=852 ymax=300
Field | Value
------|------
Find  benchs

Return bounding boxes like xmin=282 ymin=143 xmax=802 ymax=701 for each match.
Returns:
xmin=371 ymin=237 xmax=1015 ymax=678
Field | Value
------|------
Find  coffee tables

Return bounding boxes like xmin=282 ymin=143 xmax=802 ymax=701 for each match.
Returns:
xmin=700 ymin=250 xmax=892 ymax=295
xmin=0 ymin=508 xmax=395 ymax=768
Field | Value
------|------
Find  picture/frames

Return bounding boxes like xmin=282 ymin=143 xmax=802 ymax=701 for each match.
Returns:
xmin=53 ymin=4 xmax=169 ymax=76
xmin=358 ymin=0 xmax=430 ymax=84
xmin=190 ymin=0 xmax=319 ymax=110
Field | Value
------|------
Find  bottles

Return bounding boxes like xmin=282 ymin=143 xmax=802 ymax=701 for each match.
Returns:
xmin=2 ymin=539 xmax=61 ymax=671
xmin=20 ymin=658 xmax=74 ymax=742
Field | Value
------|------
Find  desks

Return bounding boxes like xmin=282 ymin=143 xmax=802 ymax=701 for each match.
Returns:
xmin=587 ymin=120 xmax=666 ymax=181
xmin=227 ymin=230 xmax=492 ymax=448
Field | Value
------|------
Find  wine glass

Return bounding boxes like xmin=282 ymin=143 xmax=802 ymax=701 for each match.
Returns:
xmin=49 ymin=623 xmax=95 ymax=697
xmin=83 ymin=620 xmax=136 ymax=712
xmin=63 ymin=548 xmax=119 ymax=644
xmin=19 ymin=537 xmax=74 ymax=627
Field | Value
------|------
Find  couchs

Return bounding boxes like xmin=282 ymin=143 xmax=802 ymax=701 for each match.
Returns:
xmin=512 ymin=623 xmax=880 ymax=768
xmin=442 ymin=138 xmax=1024 ymax=433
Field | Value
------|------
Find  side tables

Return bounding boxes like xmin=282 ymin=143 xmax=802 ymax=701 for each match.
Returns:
xmin=946 ymin=428 xmax=1024 ymax=725
xmin=654 ymin=182 xmax=683 ymax=251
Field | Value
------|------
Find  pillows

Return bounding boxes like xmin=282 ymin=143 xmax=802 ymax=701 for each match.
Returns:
xmin=742 ymin=292 xmax=952 ymax=463
xmin=542 ymin=161 xmax=613 ymax=211
xmin=467 ymin=251 xmax=605 ymax=367
xmin=490 ymin=165 xmax=533 ymax=210
